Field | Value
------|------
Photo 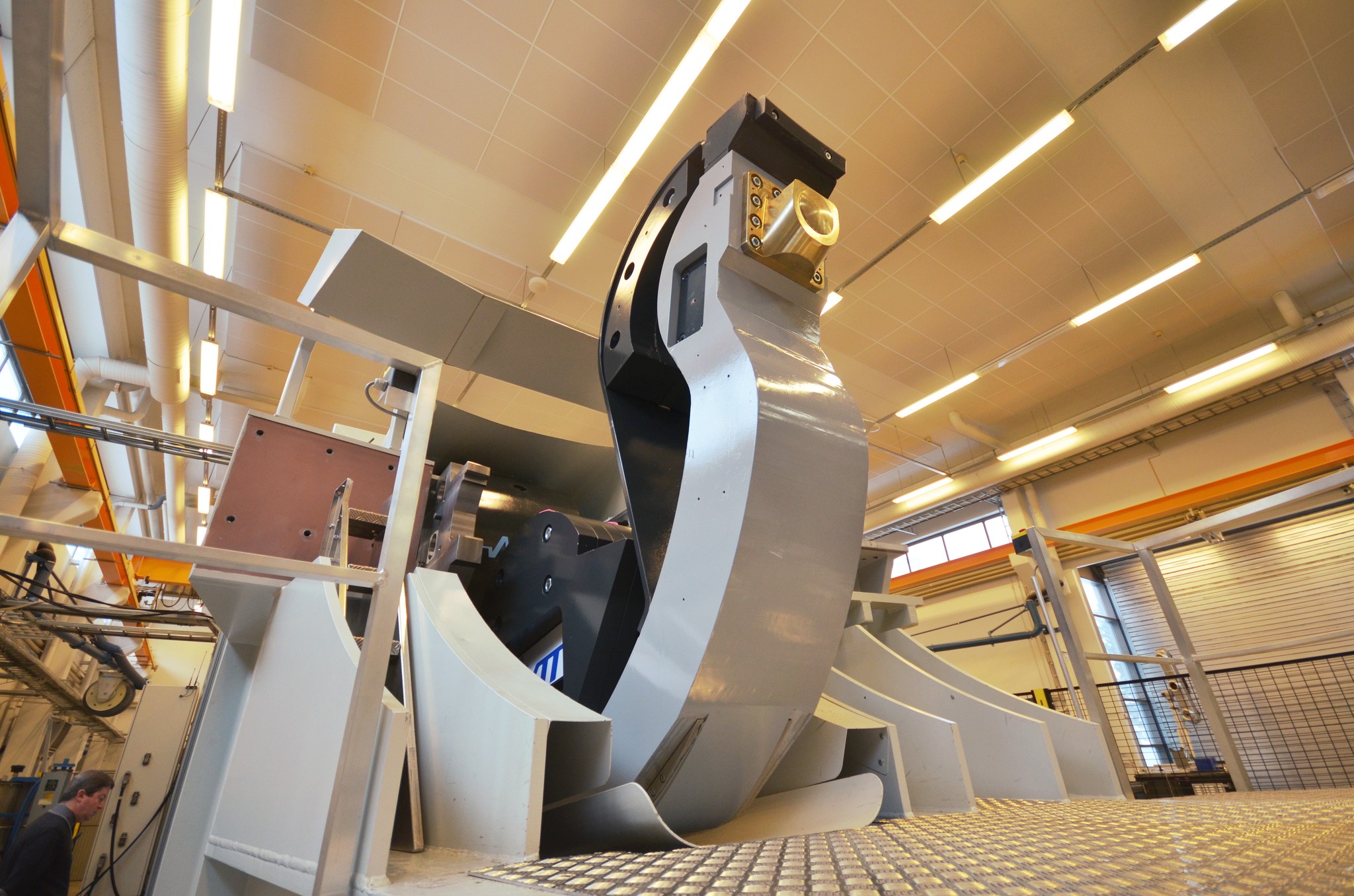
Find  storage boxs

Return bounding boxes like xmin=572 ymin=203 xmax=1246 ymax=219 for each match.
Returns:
xmin=1194 ymin=757 xmax=1219 ymax=770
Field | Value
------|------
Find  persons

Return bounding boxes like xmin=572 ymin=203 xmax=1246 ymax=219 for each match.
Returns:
xmin=0 ymin=769 xmax=114 ymax=896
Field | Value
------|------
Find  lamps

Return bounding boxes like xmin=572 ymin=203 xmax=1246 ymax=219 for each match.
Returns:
xmin=539 ymin=1 xmax=753 ymax=279
xmin=196 ymin=0 xmax=244 ymax=548
xmin=821 ymin=1 xmax=1354 ymax=514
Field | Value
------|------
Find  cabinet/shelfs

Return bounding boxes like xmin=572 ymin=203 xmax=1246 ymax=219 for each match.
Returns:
xmin=1133 ymin=771 xmax=1236 ymax=799
